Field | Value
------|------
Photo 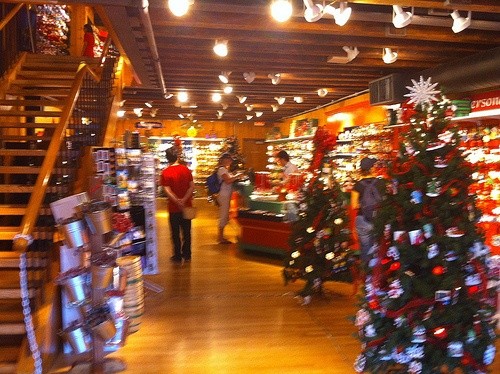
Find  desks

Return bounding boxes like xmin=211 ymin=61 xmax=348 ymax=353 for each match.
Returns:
xmin=231 ymin=217 xmax=292 ymax=258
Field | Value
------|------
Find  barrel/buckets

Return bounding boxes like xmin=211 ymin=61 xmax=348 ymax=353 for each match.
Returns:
xmin=110 ymin=319 xmax=131 ymax=346
xmin=114 ymin=257 xmax=147 ymax=332
xmin=85 ymin=311 xmax=117 ymax=341
xmin=56 ymin=269 xmax=92 ymax=305
xmin=84 ymin=202 xmax=115 ymax=235
xmin=90 ymin=264 xmax=120 ymax=292
xmin=63 ymin=219 xmax=89 ymax=250
xmin=66 ymin=323 xmax=94 ymax=353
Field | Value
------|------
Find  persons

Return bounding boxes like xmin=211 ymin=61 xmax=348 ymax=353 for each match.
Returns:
xmin=271 ymin=150 xmax=298 ymax=186
xmin=350 ymin=158 xmax=390 ymax=274
xmin=213 ymin=152 xmax=244 ymax=245
xmin=159 ymin=146 xmax=195 ymax=265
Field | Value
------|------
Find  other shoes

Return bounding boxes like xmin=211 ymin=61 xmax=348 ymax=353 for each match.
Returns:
xmin=217 ymin=239 xmax=232 ymax=244
xmin=181 ymin=254 xmax=191 ymax=261
xmin=169 ymin=255 xmax=182 ymax=261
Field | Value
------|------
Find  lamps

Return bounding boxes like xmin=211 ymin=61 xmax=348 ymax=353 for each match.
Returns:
xmin=116 ymin=0 xmax=471 ymax=121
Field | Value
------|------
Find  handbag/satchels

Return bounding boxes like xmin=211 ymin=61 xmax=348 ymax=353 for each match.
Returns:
xmin=182 ymin=207 xmax=196 ymax=219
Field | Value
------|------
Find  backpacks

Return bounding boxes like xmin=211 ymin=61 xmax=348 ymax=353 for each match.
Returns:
xmin=207 ymin=166 xmax=224 ymax=194
xmin=361 ymin=179 xmax=382 ymax=223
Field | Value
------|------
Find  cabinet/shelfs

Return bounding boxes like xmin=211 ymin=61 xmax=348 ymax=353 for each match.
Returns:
xmin=36 ymin=4 xmax=71 ymax=49
xmin=264 ymin=134 xmax=359 ymax=170
xmin=145 ymin=136 xmax=226 ymax=198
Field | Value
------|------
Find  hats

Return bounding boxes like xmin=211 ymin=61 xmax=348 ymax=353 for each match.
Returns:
xmin=360 ymin=157 xmax=377 ymax=171
